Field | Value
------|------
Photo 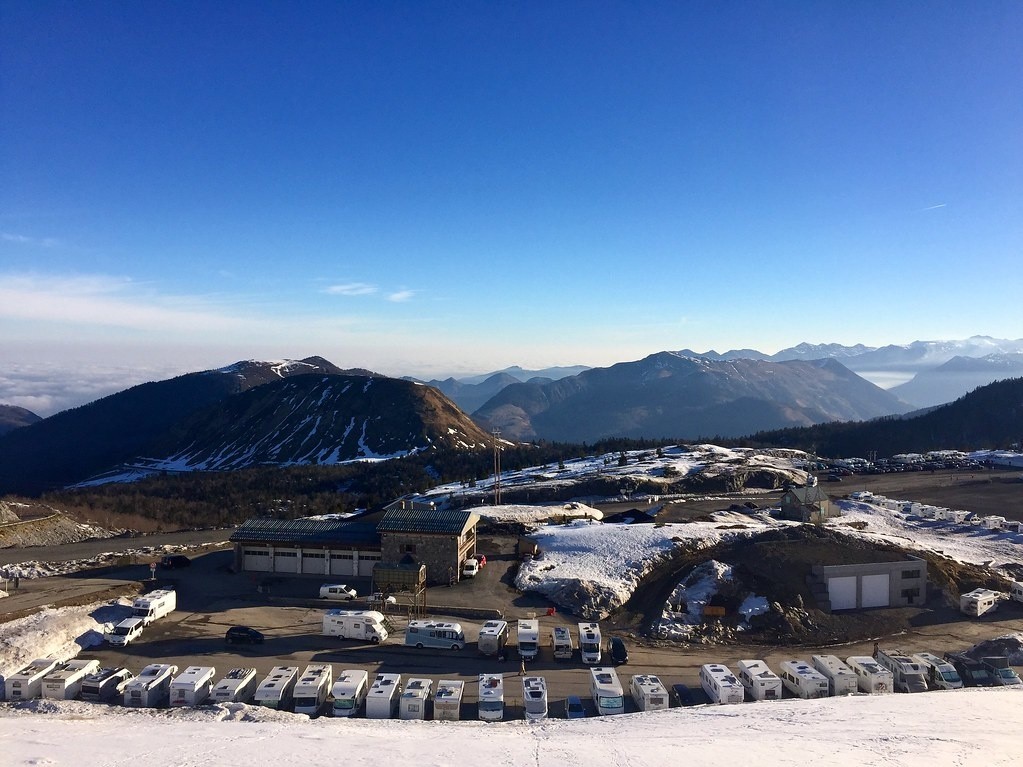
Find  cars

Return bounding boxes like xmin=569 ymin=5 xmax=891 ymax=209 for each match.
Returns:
xmin=366 ymin=595 xmax=397 ymax=604
xmin=225 ymin=627 xmax=265 ymax=643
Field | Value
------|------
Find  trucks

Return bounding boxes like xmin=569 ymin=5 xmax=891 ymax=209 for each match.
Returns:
xmin=479 ymin=674 xmax=507 ymax=722
xmin=780 ymin=660 xmax=830 ymax=698
xmin=5 ymin=659 xmax=64 ymax=702
xmin=913 ymin=652 xmax=965 ymax=689
xmin=812 ymin=654 xmax=859 ymax=697
xmin=133 ymin=589 xmax=177 ymax=627
xmin=42 ymin=661 xmax=103 ymax=701
xmin=400 ymin=678 xmax=434 ymax=720
xmin=210 ymin=668 xmax=257 ymax=705
xmin=523 ymin=676 xmax=551 ymax=720
xmin=842 ymin=656 xmax=895 ymax=693
xmin=961 ymin=588 xmax=1000 ymax=619
xmin=323 ymin=610 xmax=388 ymax=643
xmin=332 ymin=669 xmax=369 ymax=718
xmin=738 ymin=659 xmax=783 ymax=700
xmin=292 ymin=664 xmax=333 ymax=717
xmin=255 ymin=666 xmax=300 ymax=711
xmin=123 ymin=663 xmax=179 ymax=708
xmin=434 ymin=680 xmax=466 ymax=720
xmin=700 ymin=664 xmax=746 ymax=702
xmin=170 ymin=666 xmax=217 ymax=706
xmin=980 ymin=657 xmax=1022 ymax=686
xmin=589 ymin=666 xmax=627 ymax=715
xmin=879 ymin=649 xmax=930 ymax=692
xmin=366 ymin=673 xmax=402 ymax=719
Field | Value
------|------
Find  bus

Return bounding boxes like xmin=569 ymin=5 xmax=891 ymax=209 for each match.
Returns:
xmin=403 ymin=621 xmax=465 ymax=650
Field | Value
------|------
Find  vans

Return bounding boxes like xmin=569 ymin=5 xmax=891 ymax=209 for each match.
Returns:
xmin=579 ymin=622 xmax=603 ymax=664
xmin=630 ymin=674 xmax=670 ymax=710
xmin=478 ymin=621 xmax=512 ymax=654
xmin=80 ymin=666 xmax=134 ymax=703
xmin=550 ymin=628 xmax=577 ymax=656
xmin=943 ymin=653 xmax=994 ymax=688
xmin=110 ymin=618 xmax=146 ymax=646
xmin=462 ymin=558 xmax=479 ymax=578
xmin=517 ymin=618 xmax=540 ymax=657
xmin=1010 ymin=582 xmax=1023 ymax=602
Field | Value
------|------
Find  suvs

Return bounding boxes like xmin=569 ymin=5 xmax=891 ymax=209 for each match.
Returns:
xmin=566 ymin=695 xmax=586 ymax=719
xmin=474 ymin=554 xmax=487 ymax=568
xmin=608 ymin=638 xmax=630 ymax=662
xmin=319 ymin=584 xmax=358 ymax=601
